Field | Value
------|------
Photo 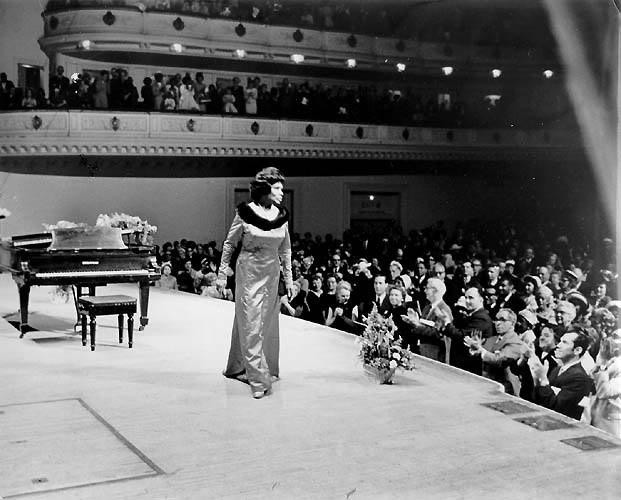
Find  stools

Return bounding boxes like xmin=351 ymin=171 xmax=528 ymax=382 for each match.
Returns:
xmin=79 ymin=295 xmax=138 ymax=351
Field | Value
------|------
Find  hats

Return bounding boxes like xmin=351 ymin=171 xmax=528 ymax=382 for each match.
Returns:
xmin=161 ymin=262 xmax=173 ymax=270
xmin=524 ymin=275 xmax=541 ymax=289
xmin=399 ymin=274 xmax=412 ymax=290
xmin=518 ymin=308 xmax=538 ymax=325
xmin=256 ymin=167 xmax=285 ymax=183
xmin=562 ymin=269 xmax=577 ymax=284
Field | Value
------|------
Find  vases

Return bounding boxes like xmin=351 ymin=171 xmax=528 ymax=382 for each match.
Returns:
xmin=362 ymin=363 xmax=396 ymax=385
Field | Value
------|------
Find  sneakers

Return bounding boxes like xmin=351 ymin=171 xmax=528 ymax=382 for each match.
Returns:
xmin=253 ymin=391 xmax=264 ymax=398
xmin=236 ymin=371 xmax=248 ymax=381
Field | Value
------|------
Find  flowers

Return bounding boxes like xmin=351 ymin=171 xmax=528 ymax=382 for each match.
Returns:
xmin=356 ymin=301 xmax=418 ymax=372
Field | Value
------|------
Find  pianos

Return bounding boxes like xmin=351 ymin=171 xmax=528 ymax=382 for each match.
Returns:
xmin=1 ymin=221 xmax=162 ymax=339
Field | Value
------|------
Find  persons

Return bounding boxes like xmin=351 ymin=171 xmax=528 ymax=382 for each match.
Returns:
xmin=41 ymin=0 xmax=557 ymax=51
xmin=146 ymin=226 xmax=619 ymax=431
xmin=1 ymin=65 xmax=571 ymax=127
xmin=217 ymin=166 xmax=297 ymax=398
